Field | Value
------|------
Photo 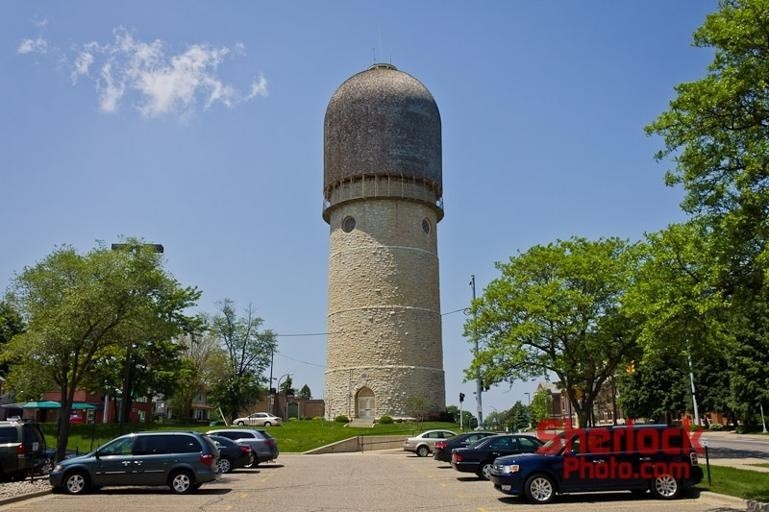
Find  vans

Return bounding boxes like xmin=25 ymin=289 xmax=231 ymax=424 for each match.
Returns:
xmin=204 ymin=429 xmax=280 ymax=468
xmin=0 ymin=414 xmax=47 ymax=484
xmin=49 ymin=431 xmax=217 ymax=495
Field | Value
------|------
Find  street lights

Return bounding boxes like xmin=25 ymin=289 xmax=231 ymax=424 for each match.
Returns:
xmin=107 ymin=237 xmax=165 ymax=427
xmin=276 ymin=371 xmax=293 ymax=416
xmin=262 ymin=340 xmax=274 ymax=415
xmin=523 ymin=392 xmax=530 ymax=404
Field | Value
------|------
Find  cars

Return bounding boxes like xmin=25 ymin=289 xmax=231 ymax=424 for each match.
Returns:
xmin=232 ymin=411 xmax=282 ymax=427
xmin=203 ymin=434 xmax=255 ymax=473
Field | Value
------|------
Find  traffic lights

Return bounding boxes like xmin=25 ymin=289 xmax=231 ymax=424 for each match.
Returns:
xmin=627 ymin=360 xmax=636 ymax=373
xmin=459 ymin=392 xmax=464 ymax=401
